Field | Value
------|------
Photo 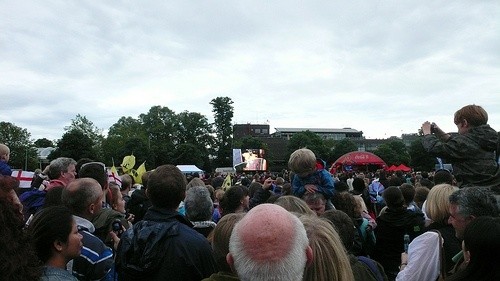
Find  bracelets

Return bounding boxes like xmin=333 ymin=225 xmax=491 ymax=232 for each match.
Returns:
xmin=399 ymin=262 xmax=408 ymax=269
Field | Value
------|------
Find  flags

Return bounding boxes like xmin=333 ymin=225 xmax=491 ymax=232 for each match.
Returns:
xmin=110 ymin=166 xmax=118 ymax=176
xmin=133 ymin=163 xmax=147 ymax=184
xmin=220 ymin=176 xmax=233 ymax=192
xmin=127 ymin=169 xmax=142 ymax=184
xmin=11 ymin=171 xmax=35 ymax=189
xmin=106 ymin=170 xmax=122 ymax=189
xmin=120 ymin=155 xmax=135 ymax=173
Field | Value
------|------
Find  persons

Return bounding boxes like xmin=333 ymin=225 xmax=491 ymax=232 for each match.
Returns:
xmin=0 ymin=105 xmax=500 ymax=281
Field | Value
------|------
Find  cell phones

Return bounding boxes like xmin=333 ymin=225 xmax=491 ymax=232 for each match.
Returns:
xmin=111 ymin=219 xmax=122 ymax=237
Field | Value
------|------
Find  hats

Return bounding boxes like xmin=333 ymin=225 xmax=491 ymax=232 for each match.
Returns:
xmin=320 ymin=209 xmax=357 ymax=232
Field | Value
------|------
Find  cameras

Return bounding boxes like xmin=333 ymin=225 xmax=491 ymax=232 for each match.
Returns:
xmin=431 ymin=124 xmax=435 ymax=132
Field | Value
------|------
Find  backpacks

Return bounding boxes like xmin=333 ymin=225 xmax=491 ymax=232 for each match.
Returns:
xmin=18 ymin=188 xmax=45 ymax=220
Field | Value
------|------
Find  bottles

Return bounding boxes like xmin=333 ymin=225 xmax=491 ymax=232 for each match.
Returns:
xmin=404 ymin=234 xmax=409 ymax=253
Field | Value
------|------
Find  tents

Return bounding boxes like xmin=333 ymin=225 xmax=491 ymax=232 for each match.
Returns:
xmin=434 ymin=164 xmax=453 ymax=173
xmin=176 ymin=164 xmax=203 ymax=180
xmin=385 ymin=165 xmax=400 ymax=176
xmin=396 ymin=164 xmax=411 ymax=173
